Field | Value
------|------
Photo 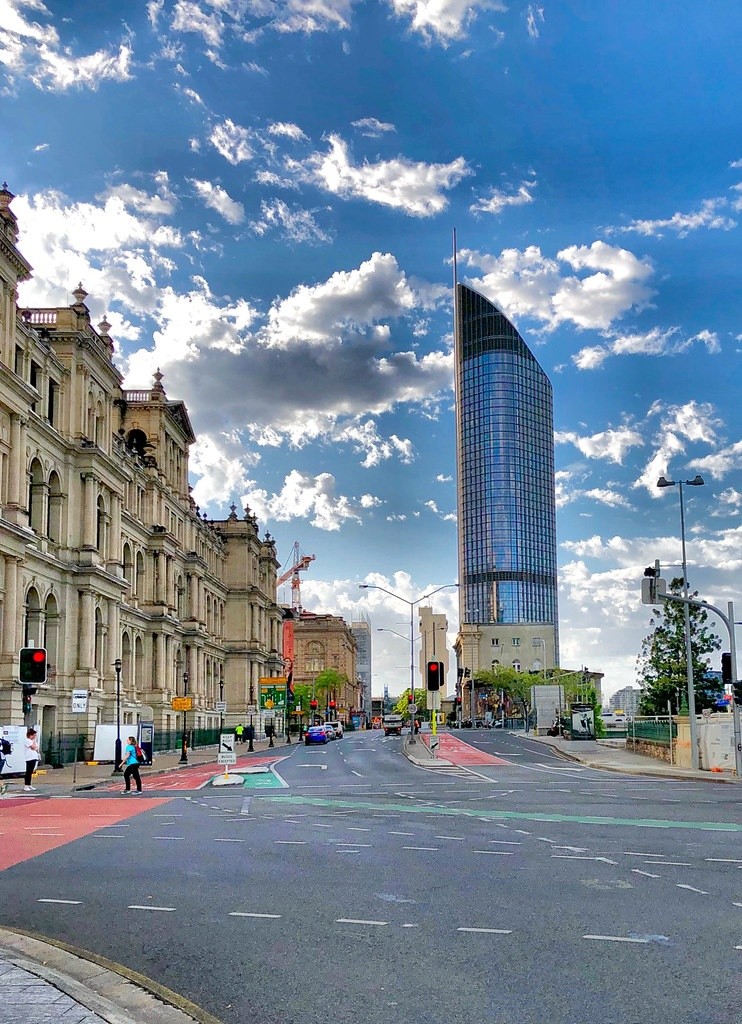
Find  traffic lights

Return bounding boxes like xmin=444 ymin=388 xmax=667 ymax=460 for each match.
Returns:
xmin=408 ymin=695 xmax=412 ymax=704
xmin=428 ymin=662 xmax=440 ymax=691
xmin=733 ymin=680 xmax=742 ymax=705
xmin=329 ymin=702 xmax=336 ymax=709
xmin=721 ymin=653 xmax=732 ymax=684
xmin=18 ymin=647 xmax=47 ymax=685
xmin=310 ymin=701 xmax=317 ymax=710
xmin=455 ymin=667 xmax=471 ymax=705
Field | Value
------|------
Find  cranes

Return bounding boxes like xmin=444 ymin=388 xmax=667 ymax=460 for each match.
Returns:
xmin=276 ymin=541 xmax=316 ymax=613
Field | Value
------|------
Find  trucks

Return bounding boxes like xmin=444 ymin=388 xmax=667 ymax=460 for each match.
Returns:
xmin=383 ymin=714 xmax=403 ymax=735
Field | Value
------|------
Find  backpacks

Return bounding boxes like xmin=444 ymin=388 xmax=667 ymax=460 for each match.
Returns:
xmin=129 ymin=743 xmax=148 ymax=762
xmin=0 ymin=738 xmax=11 ymax=755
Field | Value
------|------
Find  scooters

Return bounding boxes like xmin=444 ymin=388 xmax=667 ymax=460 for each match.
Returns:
xmin=547 ymin=715 xmax=571 ymax=737
xmin=449 ymin=717 xmax=508 ymax=729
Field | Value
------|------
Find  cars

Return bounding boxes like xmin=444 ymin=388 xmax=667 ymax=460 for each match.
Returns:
xmin=598 ymin=713 xmax=631 ymax=723
xmin=290 ymin=721 xmax=343 ymax=746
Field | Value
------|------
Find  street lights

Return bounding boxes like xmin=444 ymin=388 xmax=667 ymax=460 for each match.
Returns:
xmin=113 ymin=659 xmax=124 ymax=773
xmin=179 ymin=672 xmax=189 ymax=761
xmin=247 ymin=685 xmax=255 ymax=752
xmin=217 ymin=679 xmax=226 ymax=735
xmin=657 ymin=476 xmax=705 ymax=770
xmin=359 ymin=584 xmax=462 ymax=745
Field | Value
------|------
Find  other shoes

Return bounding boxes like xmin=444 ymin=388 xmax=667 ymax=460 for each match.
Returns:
xmin=132 ymin=790 xmax=142 ymax=795
xmin=1 ymin=785 xmax=6 ymax=795
xmin=29 ymin=785 xmax=37 ymax=790
xmin=22 ymin=786 xmax=31 ymax=791
xmin=121 ymin=789 xmax=130 ymax=794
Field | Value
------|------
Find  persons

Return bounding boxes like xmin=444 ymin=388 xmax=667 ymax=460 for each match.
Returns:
xmin=414 ymin=719 xmax=422 ymax=734
xmin=235 ymin=724 xmax=244 ymax=745
xmin=0 ymin=736 xmax=8 ymax=795
xmin=24 ymin=729 xmax=41 ymax=791
xmin=302 ymin=722 xmax=308 ymax=735
xmin=118 ymin=737 xmax=142 ymax=794
